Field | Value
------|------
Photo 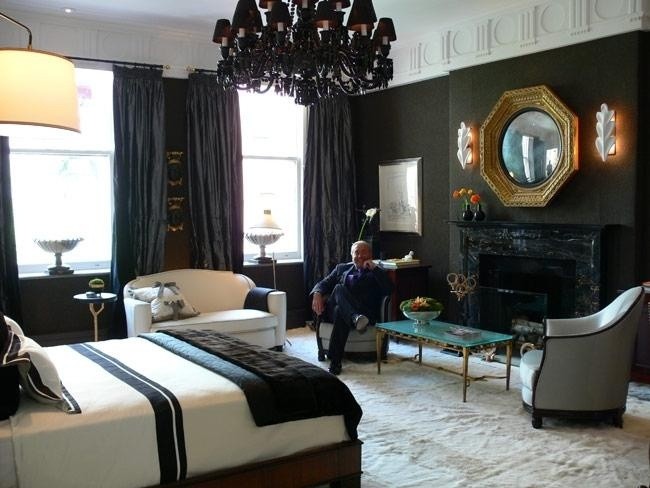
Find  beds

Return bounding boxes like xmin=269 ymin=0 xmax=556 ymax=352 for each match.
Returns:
xmin=0 ymin=315 xmax=363 ymax=488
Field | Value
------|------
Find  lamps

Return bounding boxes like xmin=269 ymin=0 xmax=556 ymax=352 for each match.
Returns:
xmin=0 ymin=11 xmax=80 ymax=136
xmin=212 ymin=0 xmax=397 ymax=107
xmin=595 ymin=103 xmax=617 ymax=163
xmin=457 ymin=122 xmax=473 ymax=170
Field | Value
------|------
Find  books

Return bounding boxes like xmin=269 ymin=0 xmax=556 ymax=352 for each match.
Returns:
xmin=445 ymin=328 xmax=480 ymax=339
xmin=379 ymin=259 xmax=421 ymax=266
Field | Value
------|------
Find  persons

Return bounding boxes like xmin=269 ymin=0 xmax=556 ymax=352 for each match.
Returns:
xmin=309 ymin=240 xmax=396 ymax=375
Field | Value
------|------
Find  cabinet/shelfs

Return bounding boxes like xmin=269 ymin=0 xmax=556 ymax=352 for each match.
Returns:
xmin=370 ymin=262 xmax=433 ymax=322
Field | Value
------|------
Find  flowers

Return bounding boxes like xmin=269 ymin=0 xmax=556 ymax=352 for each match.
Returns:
xmin=470 ymin=194 xmax=482 ymax=205
xmin=453 ymin=187 xmax=473 ymax=205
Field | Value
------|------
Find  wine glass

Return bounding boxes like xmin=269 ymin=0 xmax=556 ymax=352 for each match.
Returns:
xmin=404 ymin=312 xmax=443 ymax=326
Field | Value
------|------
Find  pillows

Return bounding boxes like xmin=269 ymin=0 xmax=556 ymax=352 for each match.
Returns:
xmin=127 ymin=281 xmax=200 ymax=323
xmin=3 ymin=314 xmax=82 ymax=414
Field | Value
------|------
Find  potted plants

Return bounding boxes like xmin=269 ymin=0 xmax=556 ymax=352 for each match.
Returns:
xmin=400 ymin=297 xmax=444 ymax=326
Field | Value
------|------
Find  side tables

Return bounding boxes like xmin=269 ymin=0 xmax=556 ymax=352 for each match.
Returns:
xmin=74 ymin=293 xmax=118 ymax=341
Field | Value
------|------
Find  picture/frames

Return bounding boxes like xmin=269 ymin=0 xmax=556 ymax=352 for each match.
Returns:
xmin=375 ymin=157 xmax=422 ymax=237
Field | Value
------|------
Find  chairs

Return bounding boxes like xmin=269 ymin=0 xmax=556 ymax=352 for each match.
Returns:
xmin=520 ymin=286 xmax=645 ymax=429
xmin=123 ymin=269 xmax=288 ymax=350
xmin=316 ymin=295 xmax=391 ymax=364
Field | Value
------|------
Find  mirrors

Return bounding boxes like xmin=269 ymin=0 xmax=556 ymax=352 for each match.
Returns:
xmin=502 ymin=110 xmax=562 ymax=185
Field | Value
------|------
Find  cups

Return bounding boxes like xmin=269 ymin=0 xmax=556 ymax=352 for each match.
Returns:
xmin=86 ymin=292 xmax=95 ymax=298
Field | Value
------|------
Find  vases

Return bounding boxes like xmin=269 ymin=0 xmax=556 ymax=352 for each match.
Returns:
xmin=462 ymin=205 xmax=473 ymax=221
xmin=474 ymin=204 xmax=485 ymax=221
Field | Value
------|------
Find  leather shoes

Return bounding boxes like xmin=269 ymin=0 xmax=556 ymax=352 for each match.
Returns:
xmin=328 ymin=361 xmax=342 ymax=375
xmin=352 ymin=312 xmax=369 ymax=335
